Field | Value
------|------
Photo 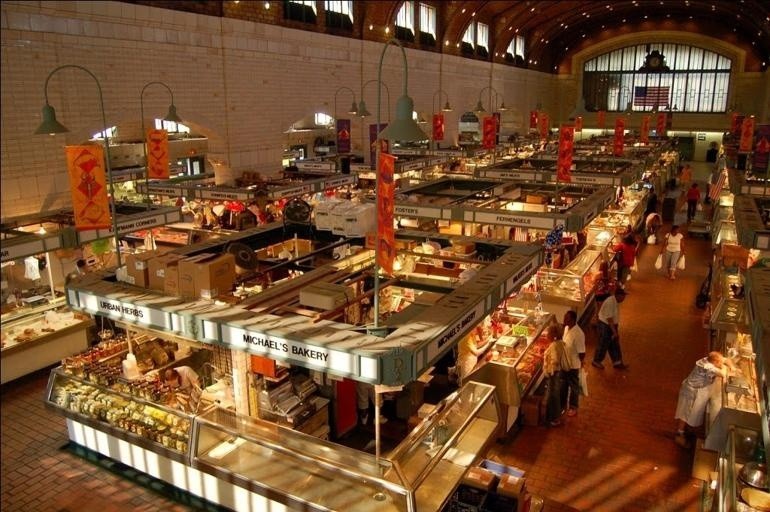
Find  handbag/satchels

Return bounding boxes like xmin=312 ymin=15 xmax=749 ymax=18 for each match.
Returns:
xmin=695 ymin=293 xmax=707 ymax=310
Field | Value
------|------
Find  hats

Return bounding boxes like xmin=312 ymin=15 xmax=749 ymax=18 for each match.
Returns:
xmin=614 ymin=288 xmax=630 ymax=296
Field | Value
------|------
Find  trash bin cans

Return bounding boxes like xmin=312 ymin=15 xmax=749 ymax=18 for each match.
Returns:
xmin=402 ymin=382 xmax=423 ymax=415
xmin=478 ymin=459 xmax=525 ymax=478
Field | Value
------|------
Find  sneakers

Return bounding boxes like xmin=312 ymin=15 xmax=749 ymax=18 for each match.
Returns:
xmin=544 ymin=420 xmax=561 ymax=429
xmin=591 ymin=360 xmax=605 ymax=369
xmin=667 ymin=274 xmax=676 ymax=280
xmin=674 ymin=429 xmax=692 ymax=451
xmin=613 ymin=360 xmax=630 ymax=369
xmin=561 ymin=408 xmax=578 ymax=416
xmin=615 ymin=278 xmax=633 ymax=289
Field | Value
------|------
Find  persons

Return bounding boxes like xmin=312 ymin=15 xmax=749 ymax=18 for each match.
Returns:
xmin=560 ymin=309 xmax=586 ymax=416
xmin=612 ymin=227 xmax=639 ymax=294
xmin=454 ymin=328 xmax=493 ymax=379
xmin=646 ymin=188 xmax=658 ymax=216
xmin=679 ymin=163 xmax=691 ymax=195
xmin=424 ymin=364 xmax=460 ymax=402
xmin=704 ymin=172 xmax=712 ymax=204
xmin=684 ymin=182 xmax=702 ymax=222
xmin=674 ymin=350 xmax=738 ymax=451
xmin=542 ymin=322 xmax=571 ymax=425
xmin=660 ymin=224 xmax=687 ymax=281
xmin=357 ymin=381 xmax=388 ymax=426
xmin=164 ymin=365 xmax=203 ymax=393
xmin=72 ymin=258 xmax=89 ymax=277
xmin=592 ymin=287 xmax=630 ymax=370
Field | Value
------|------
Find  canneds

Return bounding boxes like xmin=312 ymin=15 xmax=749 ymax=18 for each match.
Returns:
xmin=73 ymin=333 xmax=189 ymax=453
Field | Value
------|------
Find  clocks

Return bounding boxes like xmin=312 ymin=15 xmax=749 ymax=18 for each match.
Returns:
xmin=638 ymin=49 xmax=672 ymax=72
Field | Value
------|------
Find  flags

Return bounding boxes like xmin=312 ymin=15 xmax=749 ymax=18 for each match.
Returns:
xmin=142 ymin=125 xmax=171 ymax=180
xmin=334 ymin=118 xmax=351 ymax=153
xmin=597 ymin=110 xmax=606 ymax=127
xmin=574 ymin=114 xmax=583 ymax=133
xmin=375 ymin=149 xmax=400 ymax=276
xmin=482 ymin=116 xmax=496 ymax=150
xmin=528 ymin=111 xmax=538 ymax=129
xmin=556 ymin=122 xmax=573 ymax=183
xmin=639 ymin=114 xmax=652 ymax=143
xmin=61 ymin=141 xmax=114 ymax=231
xmin=614 ymin=115 xmax=625 ymax=156
xmin=655 ymin=112 xmax=667 ymax=135
xmin=369 ymin=121 xmax=390 ymax=163
xmin=536 ymin=109 xmax=549 ymax=140
xmin=432 ymin=112 xmax=445 ymax=141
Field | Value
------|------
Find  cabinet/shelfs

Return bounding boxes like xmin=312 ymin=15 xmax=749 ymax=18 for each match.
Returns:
xmin=2 ymin=176 xmax=645 ymax=512
xmin=702 ymin=146 xmax=770 ymax=512
